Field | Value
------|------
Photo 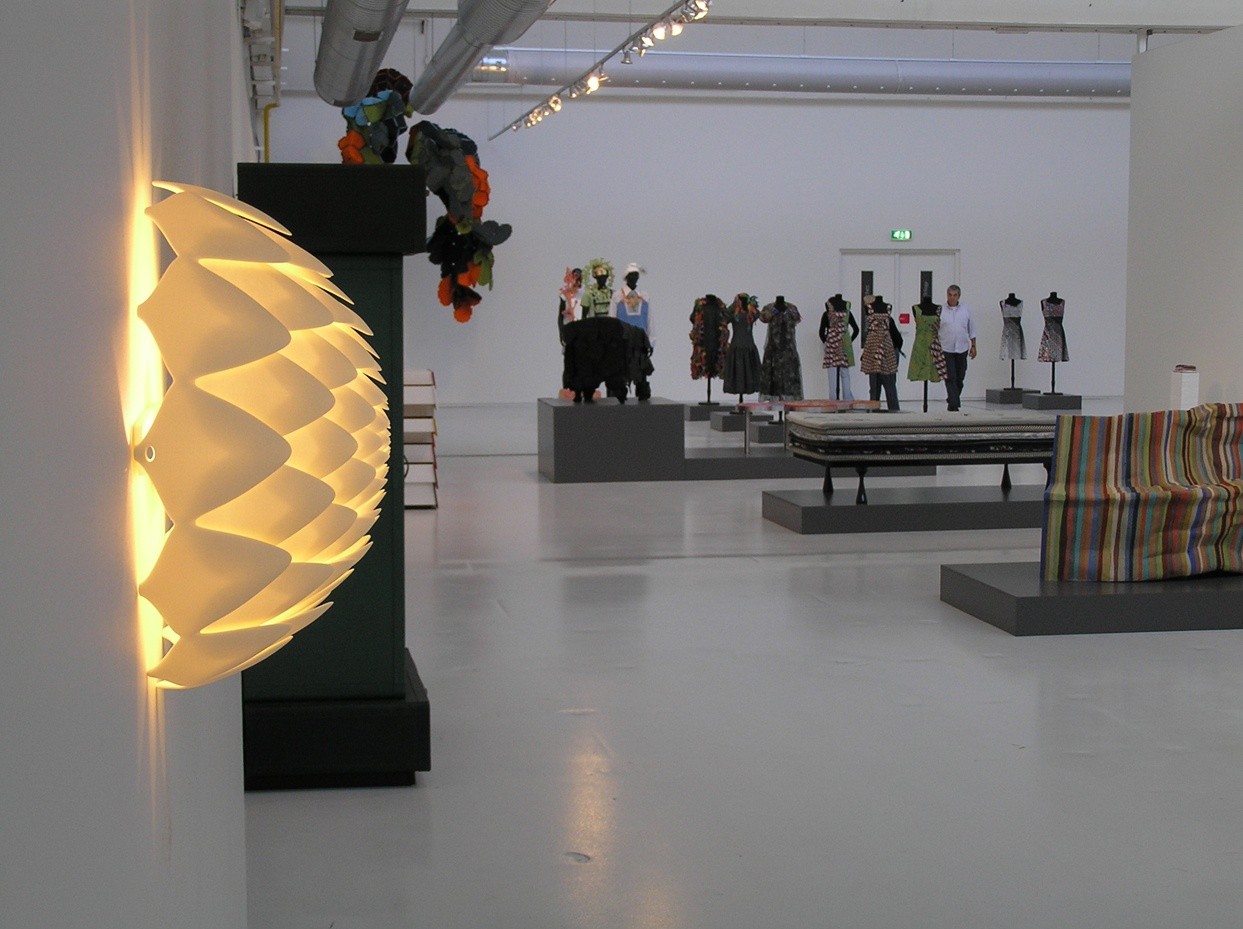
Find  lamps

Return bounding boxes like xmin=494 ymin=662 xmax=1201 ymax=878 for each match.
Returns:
xmin=505 ymin=0 xmax=713 ymax=132
xmin=131 ymin=177 xmax=393 ymax=695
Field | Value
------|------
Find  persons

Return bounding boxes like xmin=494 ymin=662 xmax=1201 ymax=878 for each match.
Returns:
xmin=1037 ymin=291 xmax=1070 ymax=362
xmin=758 ymin=296 xmax=804 ymax=401
xmin=999 ymin=292 xmax=1028 ymax=361
xmin=556 ymin=267 xmax=585 ymax=346
xmin=937 ymin=284 xmax=977 ymax=411
xmin=818 ymin=292 xmax=860 ymax=401
xmin=722 ymin=293 xmax=762 ymax=395
xmin=688 ymin=294 xmax=730 ymax=380
xmin=907 ymin=296 xmax=948 ymax=383
xmin=607 ymin=262 xmax=653 ymax=357
xmin=860 ymin=295 xmax=904 ymax=410
xmin=577 ymin=258 xmax=616 ymax=321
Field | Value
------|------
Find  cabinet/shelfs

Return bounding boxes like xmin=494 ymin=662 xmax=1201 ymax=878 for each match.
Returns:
xmin=237 ymin=156 xmax=438 ymax=795
